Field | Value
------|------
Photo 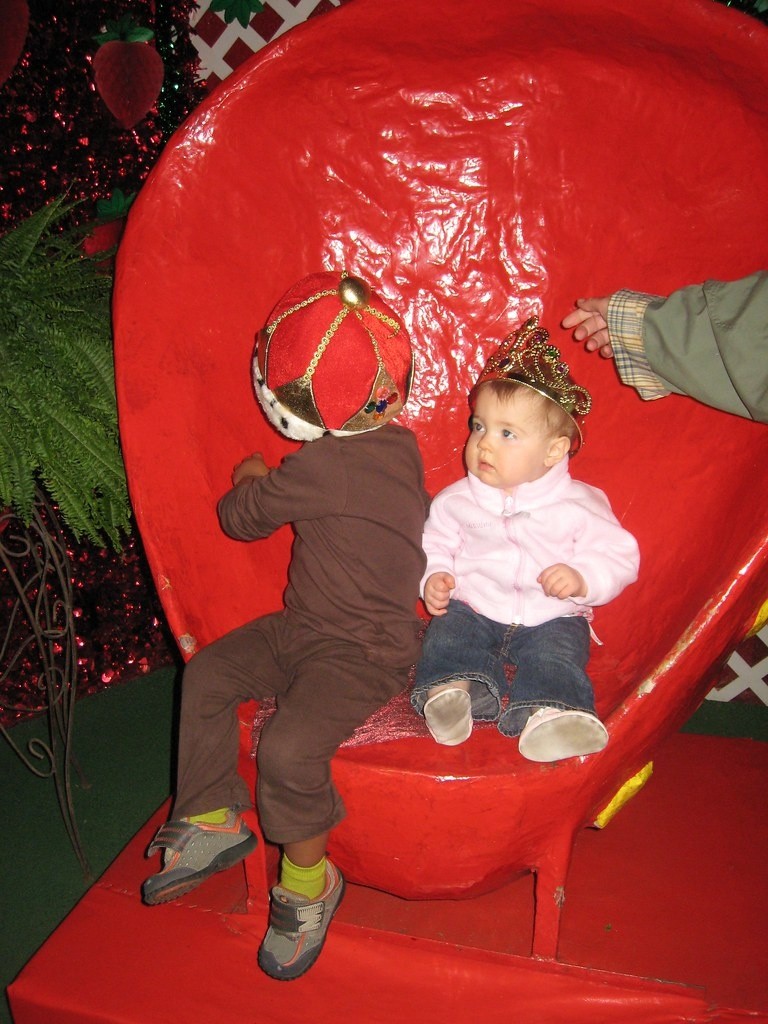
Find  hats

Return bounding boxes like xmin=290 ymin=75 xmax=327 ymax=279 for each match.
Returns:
xmin=252 ymin=270 xmax=415 ymax=440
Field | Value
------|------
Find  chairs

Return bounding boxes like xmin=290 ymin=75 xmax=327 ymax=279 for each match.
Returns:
xmin=111 ymin=0 xmax=768 ymax=958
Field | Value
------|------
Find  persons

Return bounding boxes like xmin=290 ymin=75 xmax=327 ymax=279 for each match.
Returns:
xmin=142 ymin=272 xmax=429 ymax=979
xmin=562 ymin=270 xmax=768 ymax=423
xmin=410 ymin=316 xmax=640 ymax=762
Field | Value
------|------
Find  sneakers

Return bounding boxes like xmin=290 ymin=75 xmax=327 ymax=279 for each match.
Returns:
xmin=255 ymin=859 xmax=344 ymax=983
xmin=143 ymin=809 xmax=259 ymax=905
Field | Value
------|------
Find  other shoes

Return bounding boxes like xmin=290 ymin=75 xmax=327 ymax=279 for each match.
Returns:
xmin=519 ymin=709 xmax=609 ymax=762
xmin=423 ymin=688 xmax=474 ymax=746
